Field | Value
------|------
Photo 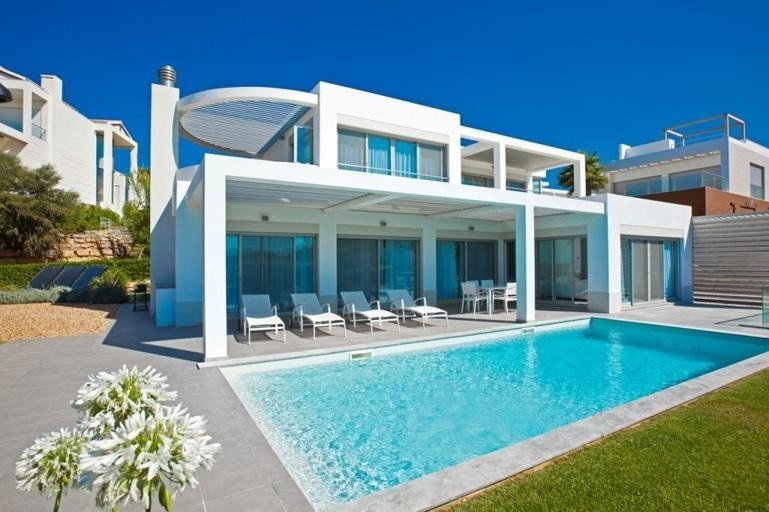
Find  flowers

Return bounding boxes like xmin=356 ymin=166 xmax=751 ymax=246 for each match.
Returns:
xmin=13 ymin=363 xmax=223 ymax=512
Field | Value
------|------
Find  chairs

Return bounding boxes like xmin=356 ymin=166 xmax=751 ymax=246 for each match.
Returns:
xmin=290 ymin=293 xmax=347 ymax=341
xmin=387 ymin=288 xmax=449 ymax=329
xmin=461 ymin=279 xmax=517 ymax=316
xmin=341 ymin=290 xmax=400 ymax=334
xmin=239 ymin=294 xmax=286 ymax=343
